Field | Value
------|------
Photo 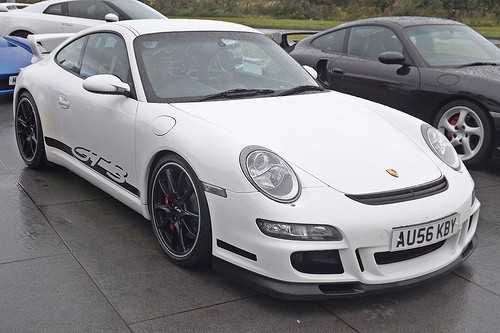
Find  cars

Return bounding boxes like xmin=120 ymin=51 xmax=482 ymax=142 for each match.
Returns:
xmin=271 ymin=16 xmax=500 ymax=168
xmin=0 ymin=0 xmax=169 ymax=96
xmin=13 ymin=18 xmax=481 ymax=301
